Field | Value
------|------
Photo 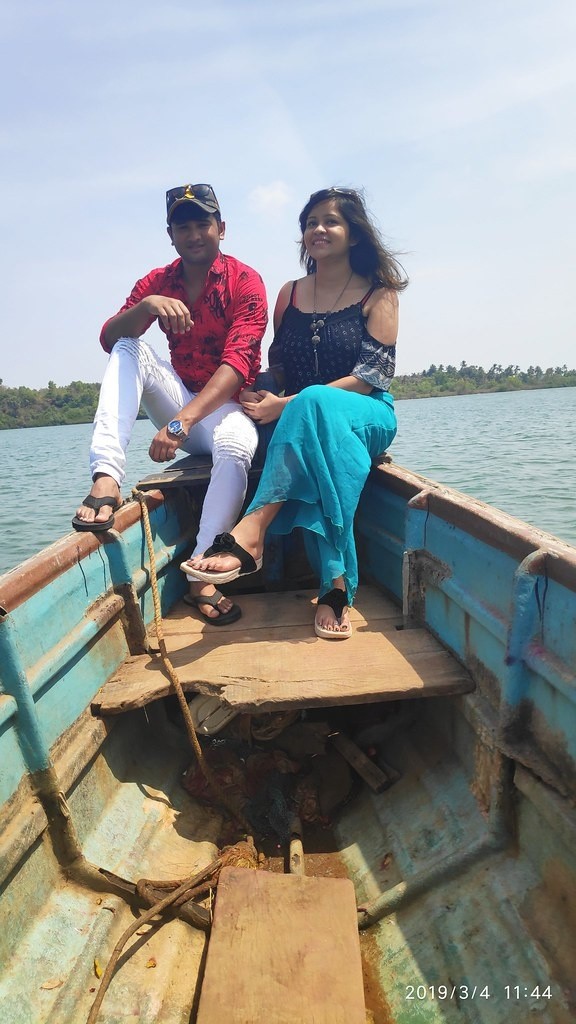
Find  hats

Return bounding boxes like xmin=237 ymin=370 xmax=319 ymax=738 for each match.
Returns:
xmin=166 ymin=185 xmax=221 ymax=222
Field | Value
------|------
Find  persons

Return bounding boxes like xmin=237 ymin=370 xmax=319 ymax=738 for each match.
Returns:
xmin=72 ymin=184 xmax=268 ymax=625
xmin=180 ymin=188 xmax=398 ymax=639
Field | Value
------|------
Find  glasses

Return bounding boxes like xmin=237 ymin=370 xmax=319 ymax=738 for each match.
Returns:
xmin=167 ymin=184 xmax=220 ymax=209
xmin=310 ymin=187 xmax=359 ymax=205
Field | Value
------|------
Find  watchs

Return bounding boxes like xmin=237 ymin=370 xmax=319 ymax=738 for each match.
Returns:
xmin=166 ymin=419 xmax=190 ymax=444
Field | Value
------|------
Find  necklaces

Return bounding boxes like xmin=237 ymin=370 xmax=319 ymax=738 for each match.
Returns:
xmin=311 ymin=270 xmax=355 ymax=376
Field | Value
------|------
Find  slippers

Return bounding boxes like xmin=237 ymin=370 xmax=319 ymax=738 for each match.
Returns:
xmin=315 ymin=587 xmax=351 ymax=639
xmin=71 ymin=493 xmax=123 ymax=531
xmin=180 ymin=533 xmax=264 ymax=585
xmin=189 ymin=585 xmax=243 ymax=626
